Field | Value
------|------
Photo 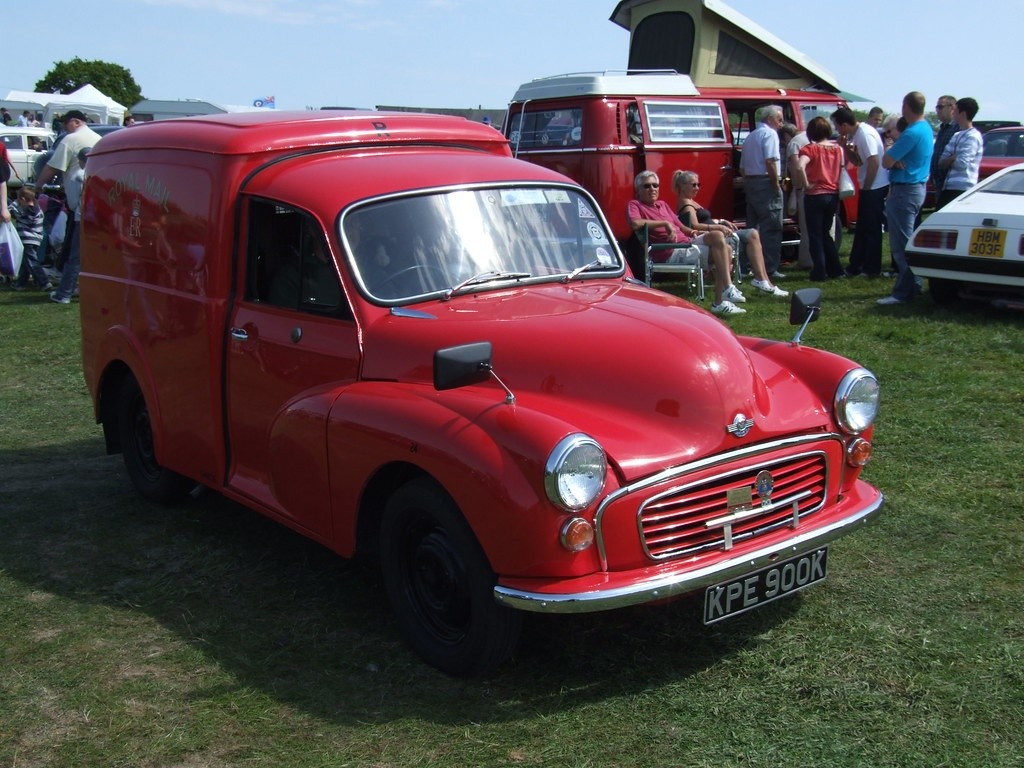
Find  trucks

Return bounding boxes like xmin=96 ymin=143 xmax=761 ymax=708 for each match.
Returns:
xmin=80 ymin=107 xmax=884 ymax=679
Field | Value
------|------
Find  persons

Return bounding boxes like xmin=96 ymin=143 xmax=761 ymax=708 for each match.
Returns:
xmin=673 ymin=170 xmax=789 ymax=297
xmin=875 ymin=91 xmax=933 ymax=305
xmin=738 ymin=94 xmax=983 ymax=282
xmin=0 ymin=106 xmax=135 ymax=306
xmin=626 ymin=170 xmax=746 ymax=316
xmin=482 ymin=117 xmax=491 ymax=126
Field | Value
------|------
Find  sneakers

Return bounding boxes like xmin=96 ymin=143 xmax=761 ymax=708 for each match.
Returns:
xmin=750 ymin=278 xmax=775 ymax=293
xmin=721 ymin=283 xmax=747 ymax=302
xmin=771 ymin=285 xmax=789 ymax=297
xmin=770 ymin=271 xmax=786 ymax=277
xmin=50 ymin=291 xmax=71 ymax=303
xmin=711 ymin=300 xmax=747 ymax=315
xmin=876 ymin=296 xmax=906 ymax=303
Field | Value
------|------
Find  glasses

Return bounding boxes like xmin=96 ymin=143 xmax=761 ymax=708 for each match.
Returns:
xmin=883 ymin=126 xmax=896 ymax=134
xmin=936 ymin=105 xmax=951 ymax=109
xmin=682 ymin=183 xmax=700 ymax=187
xmin=641 ymin=183 xmax=659 ymax=188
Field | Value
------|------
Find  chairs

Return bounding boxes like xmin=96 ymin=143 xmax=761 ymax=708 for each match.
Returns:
xmin=987 ymin=139 xmax=1008 ymax=156
xmin=643 ymin=213 xmax=742 ymax=299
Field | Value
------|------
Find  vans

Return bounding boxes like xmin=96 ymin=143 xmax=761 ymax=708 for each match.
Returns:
xmin=500 ymin=0 xmax=861 ymax=281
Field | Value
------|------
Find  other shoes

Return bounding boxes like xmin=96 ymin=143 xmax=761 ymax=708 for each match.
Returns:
xmin=882 ymin=272 xmax=898 ymax=277
xmin=788 ymin=264 xmax=811 ymax=271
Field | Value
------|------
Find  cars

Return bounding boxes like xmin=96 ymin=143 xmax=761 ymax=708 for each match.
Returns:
xmin=905 ymin=162 xmax=1024 ymax=311
xmin=922 ymin=121 xmax=1024 ymax=209
xmin=0 ymin=124 xmax=127 ymax=189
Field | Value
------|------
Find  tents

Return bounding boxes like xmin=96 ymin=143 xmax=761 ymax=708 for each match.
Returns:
xmin=0 ymin=84 xmax=285 ymax=130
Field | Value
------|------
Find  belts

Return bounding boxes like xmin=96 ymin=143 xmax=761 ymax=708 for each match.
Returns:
xmin=745 ymin=175 xmax=769 ymax=179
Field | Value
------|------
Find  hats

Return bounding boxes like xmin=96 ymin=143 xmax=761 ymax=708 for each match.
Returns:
xmin=57 ymin=110 xmax=87 ymax=122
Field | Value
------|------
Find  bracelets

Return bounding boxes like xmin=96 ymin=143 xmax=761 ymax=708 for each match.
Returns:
xmin=690 ymin=230 xmax=698 ymax=237
xmin=707 ymin=223 xmax=710 ymax=231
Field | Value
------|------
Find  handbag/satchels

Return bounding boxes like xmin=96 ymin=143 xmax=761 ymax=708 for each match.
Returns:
xmin=0 ymin=220 xmax=24 ymax=278
xmin=838 ymin=146 xmax=855 ymax=198
